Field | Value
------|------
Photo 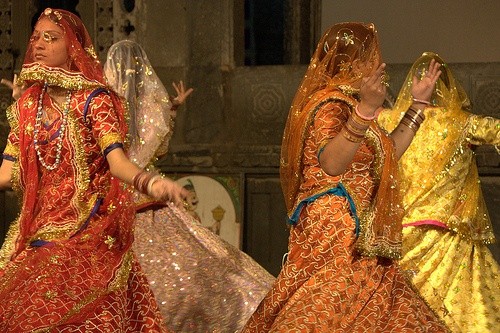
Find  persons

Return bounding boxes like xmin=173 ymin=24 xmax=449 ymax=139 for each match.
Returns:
xmin=0 ymin=40 xmax=278 ymax=333
xmin=374 ymin=52 xmax=499 ymax=333
xmin=0 ymin=8 xmax=194 ymax=333
xmin=243 ymin=21 xmax=452 ymax=333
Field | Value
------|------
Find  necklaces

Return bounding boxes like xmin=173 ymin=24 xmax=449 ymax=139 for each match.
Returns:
xmin=32 ymin=82 xmax=73 ymax=170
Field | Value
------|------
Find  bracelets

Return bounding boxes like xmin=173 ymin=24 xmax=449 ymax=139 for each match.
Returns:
xmin=412 ymin=99 xmax=431 ymax=106
xmin=400 ymin=105 xmax=425 ymax=133
xmin=169 ymin=96 xmax=178 ymax=111
xmin=132 ymin=171 xmax=162 ymax=197
xmin=341 ymin=104 xmax=376 ymax=143
